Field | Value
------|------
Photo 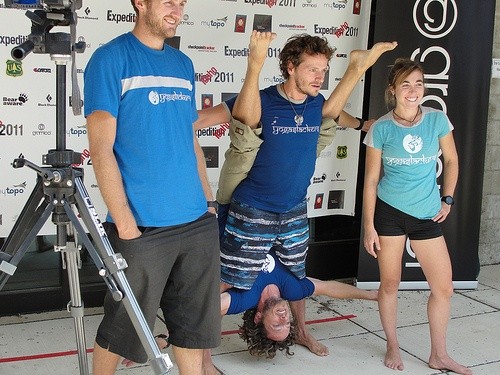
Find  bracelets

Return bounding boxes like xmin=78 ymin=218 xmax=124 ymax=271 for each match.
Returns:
xmin=153 ymin=334 xmax=170 ymax=350
xmin=354 ymin=116 xmax=364 ymax=131
xmin=206 ymin=201 xmax=219 ymax=213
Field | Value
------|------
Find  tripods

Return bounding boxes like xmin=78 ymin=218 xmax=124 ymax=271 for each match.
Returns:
xmin=0 ymin=32 xmax=172 ymax=375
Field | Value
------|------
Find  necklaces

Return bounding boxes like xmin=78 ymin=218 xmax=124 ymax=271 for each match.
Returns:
xmin=393 ymin=105 xmax=419 ymax=127
xmin=282 ymin=82 xmax=309 ymax=128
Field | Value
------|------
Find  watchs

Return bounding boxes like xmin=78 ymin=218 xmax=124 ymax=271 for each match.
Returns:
xmin=440 ymin=195 xmax=454 ymax=206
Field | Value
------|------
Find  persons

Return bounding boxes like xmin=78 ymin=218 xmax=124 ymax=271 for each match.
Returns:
xmin=83 ymin=0 xmax=222 ymax=375
xmin=121 ymin=29 xmax=398 ymax=366
xmin=362 ymin=59 xmax=471 ymax=375
